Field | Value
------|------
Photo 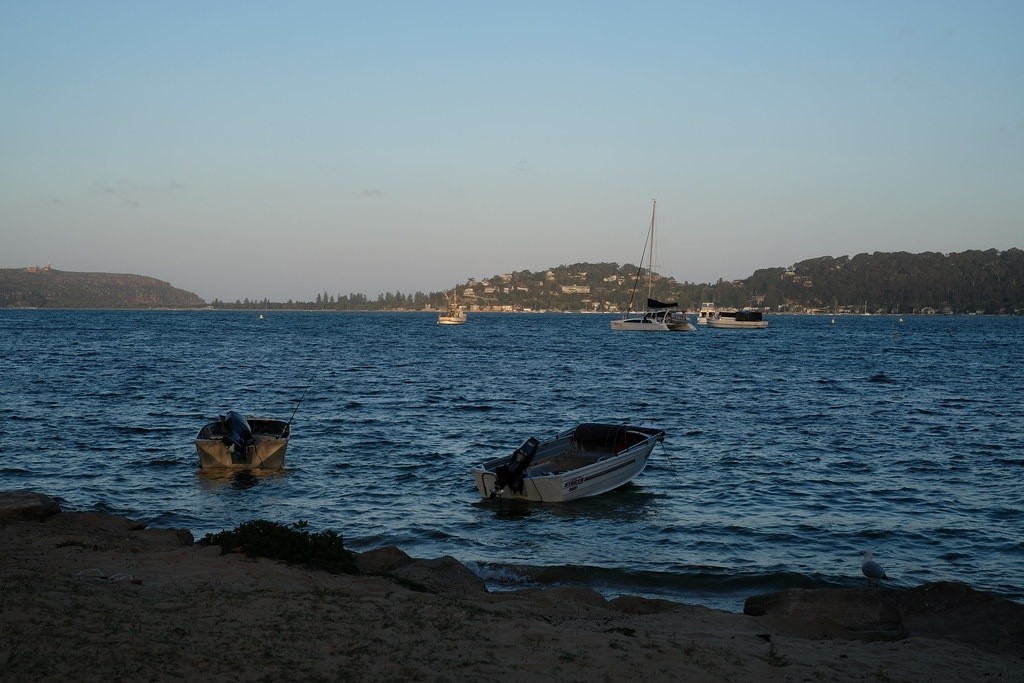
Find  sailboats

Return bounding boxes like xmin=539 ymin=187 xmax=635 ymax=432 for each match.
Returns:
xmin=609 ymin=199 xmax=698 ymax=332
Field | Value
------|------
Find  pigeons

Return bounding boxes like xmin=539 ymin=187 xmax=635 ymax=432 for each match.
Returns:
xmin=855 ymin=550 xmax=890 ymax=588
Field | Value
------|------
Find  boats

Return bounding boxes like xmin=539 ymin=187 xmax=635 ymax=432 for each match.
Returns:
xmin=436 ymin=289 xmax=467 ymax=324
xmin=194 ymin=411 xmax=290 ymax=474
xmin=472 ymin=419 xmax=667 ymax=503
xmin=697 ymin=303 xmax=769 ymax=330
